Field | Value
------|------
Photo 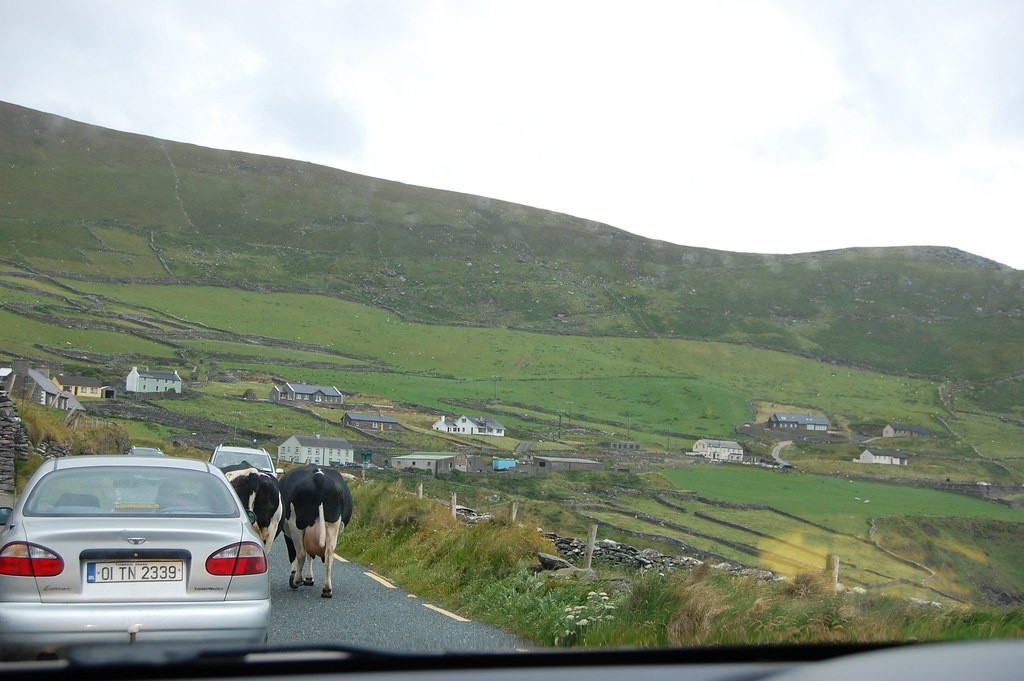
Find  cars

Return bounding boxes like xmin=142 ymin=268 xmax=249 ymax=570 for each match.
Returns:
xmin=0 ymin=455 xmax=272 ymax=661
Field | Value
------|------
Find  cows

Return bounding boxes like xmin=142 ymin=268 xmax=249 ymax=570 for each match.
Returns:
xmin=279 ymin=463 xmax=354 ymax=600
xmin=219 ymin=459 xmax=287 ymax=556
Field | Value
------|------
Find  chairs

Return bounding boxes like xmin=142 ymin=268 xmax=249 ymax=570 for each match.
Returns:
xmin=156 ymin=493 xmax=202 ymax=512
xmin=55 ymin=493 xmax=101 ymax=509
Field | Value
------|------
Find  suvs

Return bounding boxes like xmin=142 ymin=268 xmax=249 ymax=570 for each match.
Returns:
xmin=123 ymin=445 xmax=165 ymax=480
xmin=211 ymin=444 xmax=277 ymax=489
